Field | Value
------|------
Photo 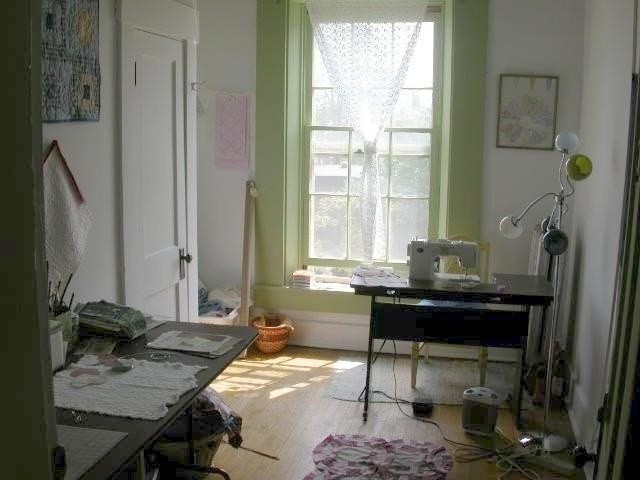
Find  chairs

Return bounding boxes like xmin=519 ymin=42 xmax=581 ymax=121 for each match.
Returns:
xmin=407 ymin=235 xmax=490 ymax=387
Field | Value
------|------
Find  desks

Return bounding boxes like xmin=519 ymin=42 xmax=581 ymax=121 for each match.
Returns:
xmin=350 ymin=266 xmax=555 ymax=429
xmin=53 ymin=320 xmax=260 ymax=480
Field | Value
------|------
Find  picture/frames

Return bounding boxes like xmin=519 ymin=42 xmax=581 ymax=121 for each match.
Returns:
xmin=496 ymin=74 xmax=559 ymax=152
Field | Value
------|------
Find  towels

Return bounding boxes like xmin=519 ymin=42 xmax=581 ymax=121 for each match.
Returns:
xmin=215 ymin=94 xmax=252 ymax=171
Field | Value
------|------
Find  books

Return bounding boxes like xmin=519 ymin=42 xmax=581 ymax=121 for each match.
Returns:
xmin=76 ymin=298 xmax=169 ymax=341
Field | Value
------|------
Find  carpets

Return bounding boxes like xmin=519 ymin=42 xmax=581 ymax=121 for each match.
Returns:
xmin=329 ymin=355 xmax=526 ymax=408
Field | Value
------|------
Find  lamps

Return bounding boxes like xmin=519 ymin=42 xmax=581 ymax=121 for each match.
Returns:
xmin=499 ymin=129 xmax=593 ymax=457
